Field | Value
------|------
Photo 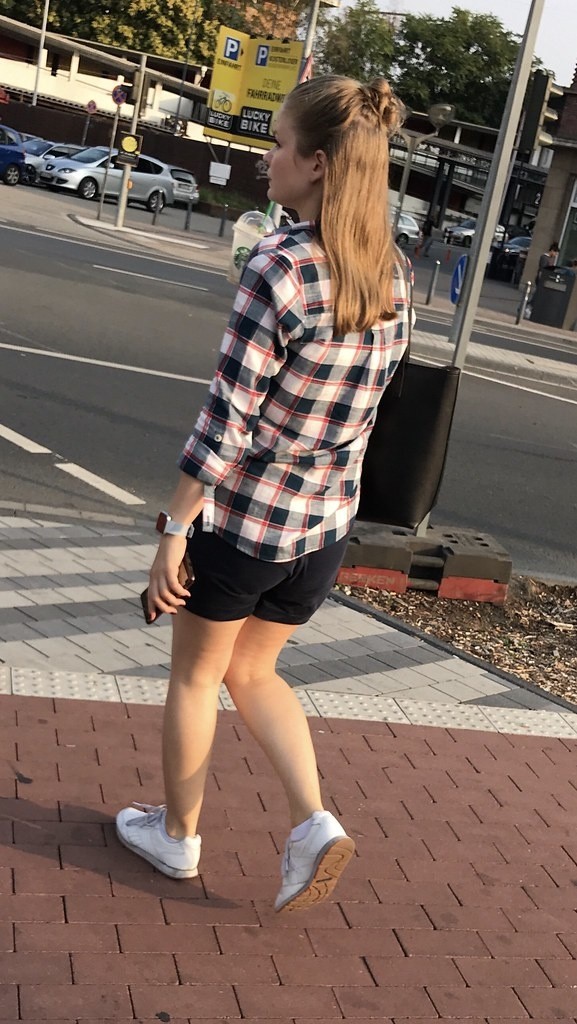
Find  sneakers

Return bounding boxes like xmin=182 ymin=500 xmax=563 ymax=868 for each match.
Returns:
xmin=274 ymin=810 xmax=355 ymax=913
xmin=115 ymin=801 xmax=202 ymax=878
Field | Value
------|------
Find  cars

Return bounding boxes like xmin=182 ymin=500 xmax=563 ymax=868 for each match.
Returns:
xmin=167 ymin=164 xmax=201 ymax=209
xmin=390 ymin=211 xmax=420 ymax=249
xmin=40 ymin=147 xmax=175 ymax=213
xmin=19 ymin=133 xmax=40 ymax=142
xmin=23 ymin=139 xmax=106 ymax=188
xmin=0 ymin=124 xmax=25 ymax=186
xmin=501 ymin=236 xmax=533 ymax=263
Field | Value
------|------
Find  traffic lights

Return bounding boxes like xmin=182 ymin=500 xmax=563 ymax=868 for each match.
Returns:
xmin=121 ymin=69 xmax=142 ymax=104
xmin=516 ymin=70 xmax=563 ymax=157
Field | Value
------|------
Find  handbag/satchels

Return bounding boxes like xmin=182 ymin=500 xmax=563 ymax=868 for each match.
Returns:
xmin=355 ymin=245 xmax=461 ymax=529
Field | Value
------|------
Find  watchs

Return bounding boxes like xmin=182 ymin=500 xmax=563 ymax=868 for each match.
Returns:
xmin=155 ymin=510 xmax=194 ymax=538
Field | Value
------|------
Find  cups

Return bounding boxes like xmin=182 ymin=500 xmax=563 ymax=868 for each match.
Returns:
xmin=227 ymin=211 xmax=275 ymax=286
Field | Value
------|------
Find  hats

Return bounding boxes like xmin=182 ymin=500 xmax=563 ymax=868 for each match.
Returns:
xmin=551 ymin=242 xmax=560 ymax=251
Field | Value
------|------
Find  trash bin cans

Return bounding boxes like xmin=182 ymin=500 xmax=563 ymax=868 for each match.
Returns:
xmin=529 ymin=265 xmax=577 ymax=330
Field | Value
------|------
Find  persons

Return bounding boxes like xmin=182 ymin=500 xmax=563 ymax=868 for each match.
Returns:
xmin=530 ymin=243 xmax=560 ymax=307
xmin=413 ymin=213 xmax=440 ymax=259
xmin=115 ymin=73 xmax=420 ymax=913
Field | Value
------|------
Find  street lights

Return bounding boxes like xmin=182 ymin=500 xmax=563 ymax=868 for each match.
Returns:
xmin=389 ymin=103 xmax=456 ymax=239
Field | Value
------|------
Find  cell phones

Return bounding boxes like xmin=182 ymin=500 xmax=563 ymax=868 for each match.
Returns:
xmin=140 ymin=553 xmax=196 ymax=624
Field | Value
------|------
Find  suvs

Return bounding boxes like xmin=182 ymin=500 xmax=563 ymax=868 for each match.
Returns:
xmin=443 ymin=218 xmax=507 ymax=248
